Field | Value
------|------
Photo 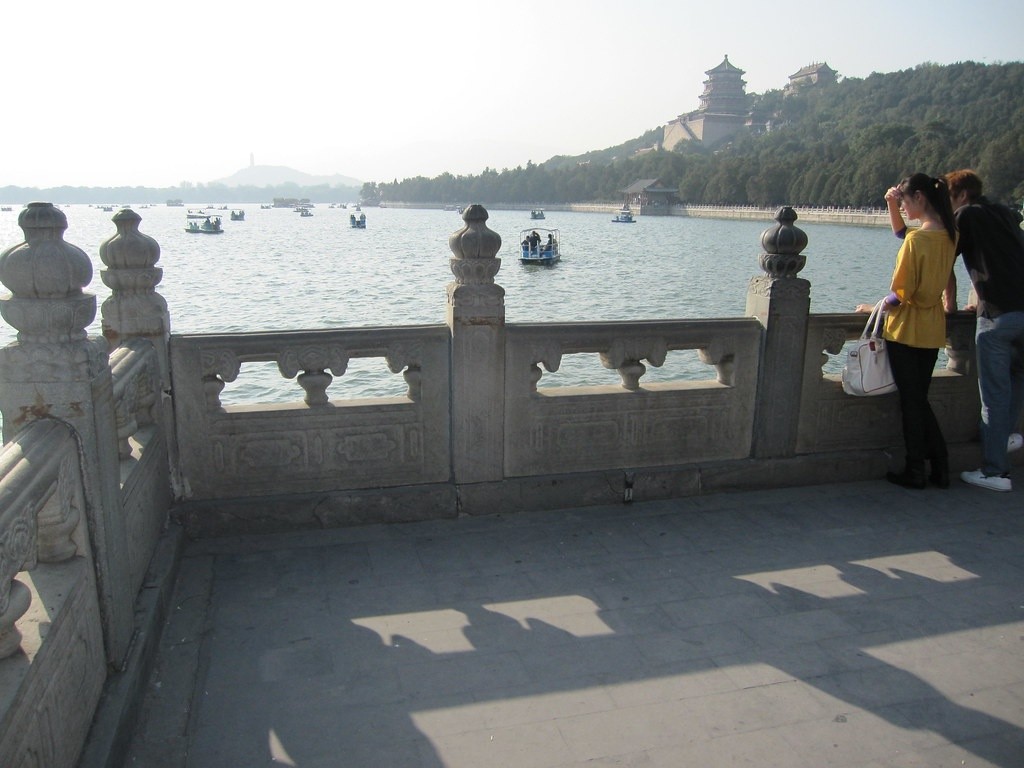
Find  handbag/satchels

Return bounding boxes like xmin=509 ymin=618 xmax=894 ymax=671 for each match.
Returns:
xmin=841 ymin=296 xmax=898 ymax=396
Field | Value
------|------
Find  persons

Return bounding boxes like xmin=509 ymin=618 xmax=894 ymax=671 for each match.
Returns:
xmin=855 ymin=172 xmax=959 ymax=489
xmin=522 ymin=230 xmax=558 ymax=250
xmin=936 ymin=168 xmax=1024 ymax=491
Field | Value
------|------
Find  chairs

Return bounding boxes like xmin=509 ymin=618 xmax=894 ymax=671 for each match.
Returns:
xmin=522 ymin=244 xmax=558 ymax=258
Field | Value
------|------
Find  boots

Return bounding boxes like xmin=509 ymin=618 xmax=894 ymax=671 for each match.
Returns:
xmin=929 ymin=458 xmax=950 ymax=488
xmin=887 ymin=455 xmax=928 ymax=490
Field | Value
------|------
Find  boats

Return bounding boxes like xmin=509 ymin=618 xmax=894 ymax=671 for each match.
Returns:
xmin=186 ymin=204 xmax=245 ymax=221
xmin=260 ymin=197 xmax=315 ymax=217
xmin=350 ymin=214 xmax=366 ymax=228
xmin=518 ymin=227 xmax=561 ymax=266
xmin=530 ymin=208 xmax=546 ymax=219
xmin=1 ymin=208 xmax=12 ymax=211
xmin=185 ymin=214 xmax=224 ymax=233
xmin=166 ymin=199 xmax=184 ymax=206
xmin=612 ymin=203 xmax=636 ymax=222
xmin=88 ymin=203 xmax=156 ymax=211
xmin=328 ymin=202 xmax=361 ymax=211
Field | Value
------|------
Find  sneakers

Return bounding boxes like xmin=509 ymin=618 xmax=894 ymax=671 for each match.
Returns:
xmin=1007 ymin=433 xmax=1023 ymax=453
xmin=960 ymin=469 xmax=1011 ymax=492
